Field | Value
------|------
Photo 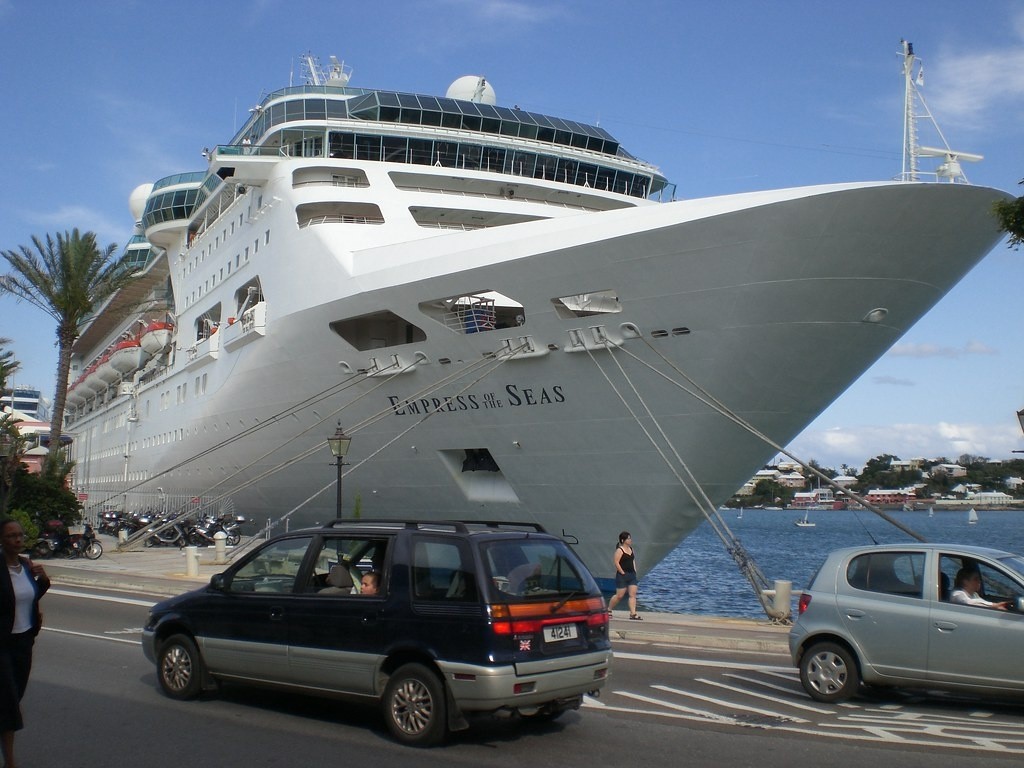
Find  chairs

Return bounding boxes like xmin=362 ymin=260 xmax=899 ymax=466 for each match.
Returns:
xmin=940 ymin=571 xmax=951 ymax=602
xmin=317 ymin=565 xmax=354 ymax=595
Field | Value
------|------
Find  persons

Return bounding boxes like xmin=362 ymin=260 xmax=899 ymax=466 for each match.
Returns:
xmin=446 ymin=548 xmax=485 ymax=599
xmin=341 ymin=546 xmax=386 ymax=594
xmin=0 ymin=519 xmax=51 ymax=768
xmin=607 ymin=531 xmax=643 ymax=620
xmin=950 ymin=566 xmax=1014 ymax=611
xmin=242 ymin=136 xmax=251 ymax=155
xmin=361 ymin=573 xmax=381 ymax=595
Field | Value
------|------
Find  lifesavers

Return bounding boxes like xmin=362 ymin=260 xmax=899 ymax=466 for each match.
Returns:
xmin=481 ymin=314 xmax=496 ymax=331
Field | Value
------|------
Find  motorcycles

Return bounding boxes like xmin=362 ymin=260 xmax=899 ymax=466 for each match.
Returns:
xmin=29 ymin=507 xmax=247 ymax=559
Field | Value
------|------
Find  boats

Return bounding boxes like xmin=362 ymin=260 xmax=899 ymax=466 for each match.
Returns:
xmin=63 ymin=354 xmax=122 ymax=410
xmin=106 ymin=341 xmax=141 ymax=373
xmin=794 ymin=510 xmax=816 ymax=527
xmin=139 ymin=321 xmax=174 ymax=355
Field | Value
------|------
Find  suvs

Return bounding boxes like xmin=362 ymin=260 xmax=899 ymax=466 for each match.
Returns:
xmin=141 ymin=518 xmax=611 ymax=748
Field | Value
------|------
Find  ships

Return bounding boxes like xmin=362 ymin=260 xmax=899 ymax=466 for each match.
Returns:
xmin=57 ymin=38 xmax=1020 ymax=603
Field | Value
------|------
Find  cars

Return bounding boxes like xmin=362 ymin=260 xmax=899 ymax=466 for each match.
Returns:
xmin=785 ymin=543 xmax=1023 ymax=712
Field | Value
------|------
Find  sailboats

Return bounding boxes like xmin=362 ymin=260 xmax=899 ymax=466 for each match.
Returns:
xmin=967 ymin=507 xmax=979 ymax=524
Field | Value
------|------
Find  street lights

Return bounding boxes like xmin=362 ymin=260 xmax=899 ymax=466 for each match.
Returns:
xmin=326 ymin=419 xmax=354 ymax=564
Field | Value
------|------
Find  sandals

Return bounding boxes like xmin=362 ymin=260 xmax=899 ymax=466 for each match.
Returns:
xmin=629 ymin=614 xmax=643 ymax=620
xmin=607 ymin=610 xmax=612 ymax=618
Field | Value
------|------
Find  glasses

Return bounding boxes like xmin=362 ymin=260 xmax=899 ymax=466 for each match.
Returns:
xmin=2 ymin=532 xmax=24 ymax=541
